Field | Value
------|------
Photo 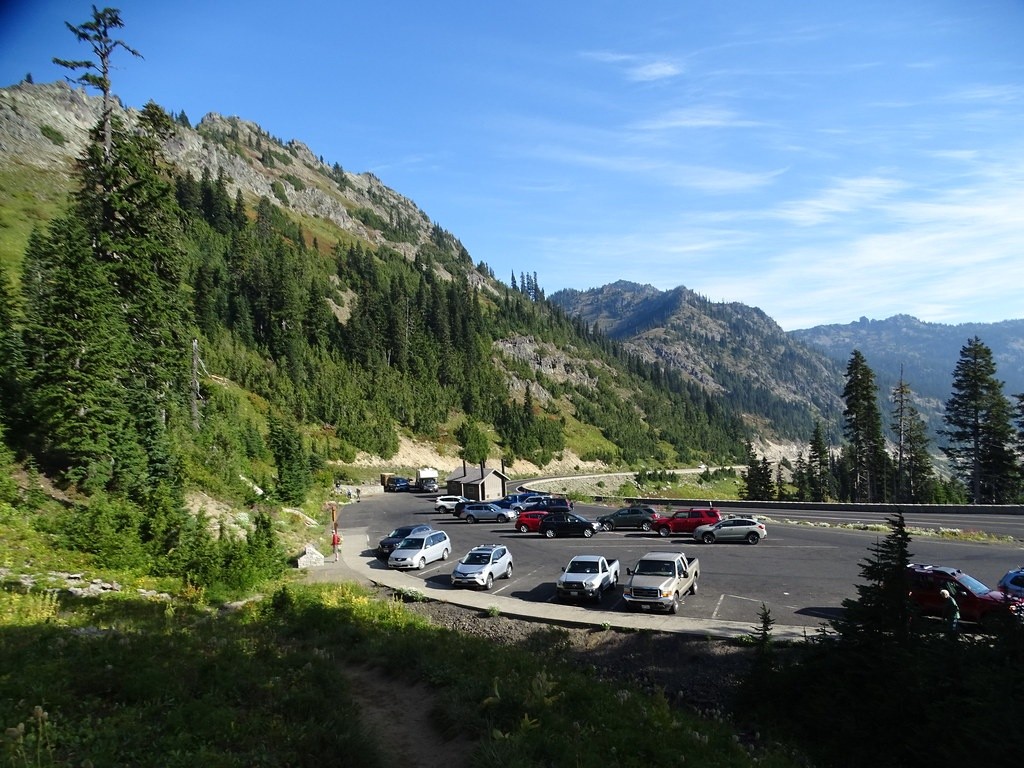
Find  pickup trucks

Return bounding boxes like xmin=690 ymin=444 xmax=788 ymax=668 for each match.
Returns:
xmin=622 ymin=550 xmax=702 ymax=614
xmin=556 ymin=555 xmax=620 ymax=604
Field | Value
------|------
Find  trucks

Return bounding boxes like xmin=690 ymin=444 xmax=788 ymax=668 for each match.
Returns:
xmin=414 ymin=468 xmax=439 ymax=488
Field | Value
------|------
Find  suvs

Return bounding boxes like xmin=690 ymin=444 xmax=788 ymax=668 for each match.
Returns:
xmin=386 ymin=529 xmax=452 ymax=570
xmin=450 ymin=543 xmax=514 ymax=590
xmin=650 ymin=508 xmax=722 ymax=537
xmin=454 ymin=491 xmax=574 ymax=523
xmin=595 ymin=506 xmax=660 ymax=532
xmin=996 ymin=565 xmax=1024 ymax=600
xmin=434 ymin=495 xmax=476 ymax=514
xmin=378 ymin=524 xmax=434 ymax=559
xmin=902 ymin=562 xmax=1024 ymax=637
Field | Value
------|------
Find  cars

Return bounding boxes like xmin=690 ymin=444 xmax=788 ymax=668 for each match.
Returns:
xmin=538 ymin=511 xmax=602 ymax=538
xmin=693 ymin=518 xmax=768 ymax=545
xmin=423 ymin=481 xmax=438 ymax=492
xmin=513 ymin=511 xmax=553 ymax=533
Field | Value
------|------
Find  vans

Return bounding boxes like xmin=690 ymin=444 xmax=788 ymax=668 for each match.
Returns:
xmin=387 ymin=477 xmax=410 ymax=492
